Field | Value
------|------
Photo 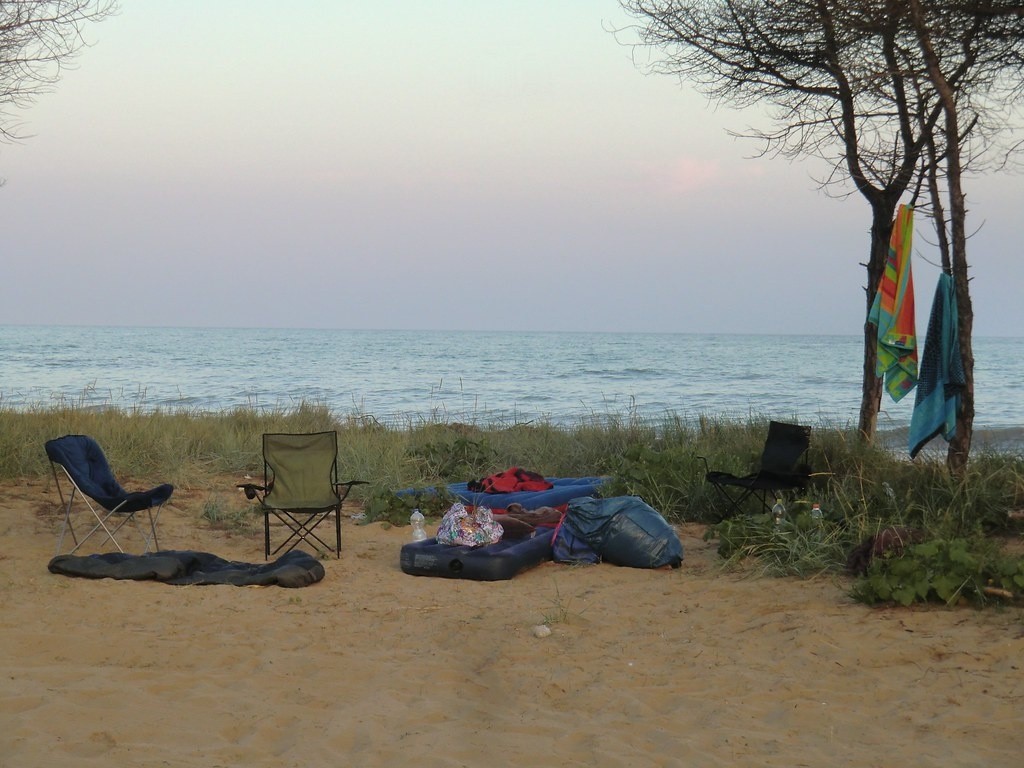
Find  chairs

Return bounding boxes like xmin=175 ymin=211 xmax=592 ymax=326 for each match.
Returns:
xmin=696 ymin=420 xmax=813 ymax=523
xmin=236 ymin=430 xmax=369 ymax=561
xmin=44 ymin=434 xmax=174 ymax=557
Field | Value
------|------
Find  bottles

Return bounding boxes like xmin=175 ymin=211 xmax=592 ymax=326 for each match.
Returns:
xmin=772 ymin=499 xmax=785 ymax=526
xmin=811 ymin=504 xmax=824 ymax=521
xmin=409 ymin=509 xmax=427 ymax=543
